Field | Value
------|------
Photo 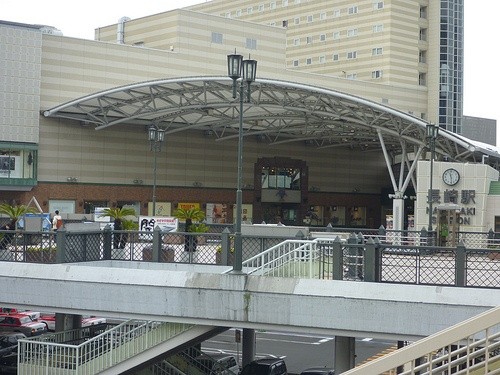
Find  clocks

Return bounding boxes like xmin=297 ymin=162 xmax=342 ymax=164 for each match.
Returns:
xmin=442 ymin=169 xmax=460 ymax=186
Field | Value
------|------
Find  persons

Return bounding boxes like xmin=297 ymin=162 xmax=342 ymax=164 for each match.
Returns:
xmin=52 ymin=210 xmax=61 ymax=243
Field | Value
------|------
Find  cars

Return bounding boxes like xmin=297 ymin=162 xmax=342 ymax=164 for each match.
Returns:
xmin=0 ymin=307 xmax=107 ymax=375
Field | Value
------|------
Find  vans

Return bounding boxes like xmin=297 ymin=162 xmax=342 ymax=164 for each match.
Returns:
xmin=299 ymin=366 xmax=335 ymax=375
xmin=192 ymin=354 xmax=240 ymax=375
xmin=239 ymin=358 xmax=288 ymax=375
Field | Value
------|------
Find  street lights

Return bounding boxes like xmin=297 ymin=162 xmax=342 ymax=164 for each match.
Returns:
xmin=422 ymin=121 xmax=441 ymax=255
xmin=148 ymin=125 xmax=165 ymax=218
xmin=225 ymin=48 xmax=258 ymax=276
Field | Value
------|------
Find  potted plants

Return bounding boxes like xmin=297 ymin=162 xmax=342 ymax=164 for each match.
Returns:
xmin=439 ymin=225 xmax=449 ymax=245
xmin=142 ymin=244 xmax=174 ymax=263
xmin=23 ymin=247 xmax=57 ymax=264
xmin=487 ymin=245 xmax=500 ymax=261
xmin=163 ymin=228 xmax=185 ymax=245
xmin=196 ymin=223 xmax=210 ymax=245
xmin=215 ymin=246 xmax=234 ymax=266
xmin=127 ymin=223 xmax=139 ymax=243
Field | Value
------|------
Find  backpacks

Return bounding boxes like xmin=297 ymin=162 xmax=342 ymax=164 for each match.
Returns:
xmin=55 ymin=218 xmax=62 ymax=228
